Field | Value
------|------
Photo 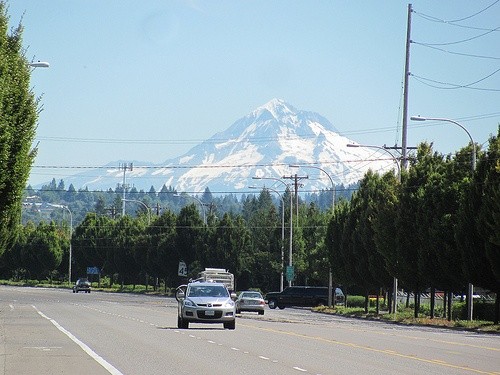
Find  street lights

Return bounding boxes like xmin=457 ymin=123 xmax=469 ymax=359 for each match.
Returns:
xmin=288 ymin=164 xmax=335 ymax=309
xmin=252 ymin=175 xmax=294 ymax=288
xmin=410 ymin=114 xmax=476 ymax=323
xmin=346 ymin=143 xmax=400 ymax=316
xmin=247 ymin=185 xmax=284 ymax=292
xmin=121 ymin=197 xmax=151 ymax=226
xmin=46 ymin=202 xmax=72 ymax=288
xmin=172 ymin=193 xmax=207 ymax=226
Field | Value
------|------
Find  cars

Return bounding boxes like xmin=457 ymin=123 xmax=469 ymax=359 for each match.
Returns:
xmin=235 ymin=292 xmax=265 ymax=316
xmin=72 ymin=280 xmax=91 ymax=293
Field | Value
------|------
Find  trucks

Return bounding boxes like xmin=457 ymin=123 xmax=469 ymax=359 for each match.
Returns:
xmin=190 ymin=267 xmax=234 ymax=297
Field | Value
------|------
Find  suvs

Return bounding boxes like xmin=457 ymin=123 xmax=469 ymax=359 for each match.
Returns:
xmin=178 ymin=283 xmax=235 ymax=329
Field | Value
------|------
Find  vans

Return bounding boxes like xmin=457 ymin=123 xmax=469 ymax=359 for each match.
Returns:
xmin=266 ymin=287 xmax=345 ymax=310
